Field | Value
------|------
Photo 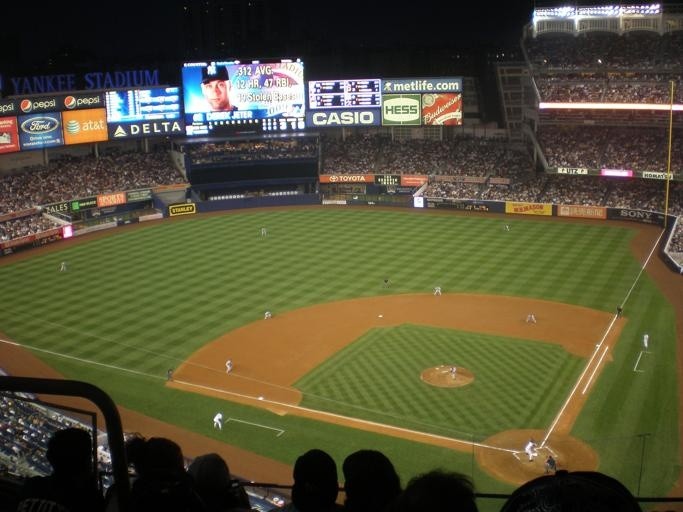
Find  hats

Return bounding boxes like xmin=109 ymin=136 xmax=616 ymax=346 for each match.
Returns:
xmin=200 ymin=65 xmax=230 ymax=85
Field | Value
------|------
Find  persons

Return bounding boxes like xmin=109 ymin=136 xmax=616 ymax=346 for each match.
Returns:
xmin=616 ymin=303 xmax=623 ymax=319
xmin=59 ymin=260 xmax=66 ymax=272
xmin=263 ymin=309 xmax=272 ymax=321
xmin=1 ymin=370 xmax=647 ymax=511
xmin=449 ymin=364 xmax=456 ymax=379
xmin=433 ymin=283 xmax=441 ymax=295
xmin=313 ymin=25 xmax=682 ymax=251
xmin=198 ymin=64 xmax=239 ymax=117
xmin=1 ymin=128 xmax=313 ymax=243
xmin=643 ymin=332 xmax=649 ymax=352
xmin=223 ymin=357 xmax=233 ymax=375
xmin=525 ymin=312 xmax=536 ymax=323
